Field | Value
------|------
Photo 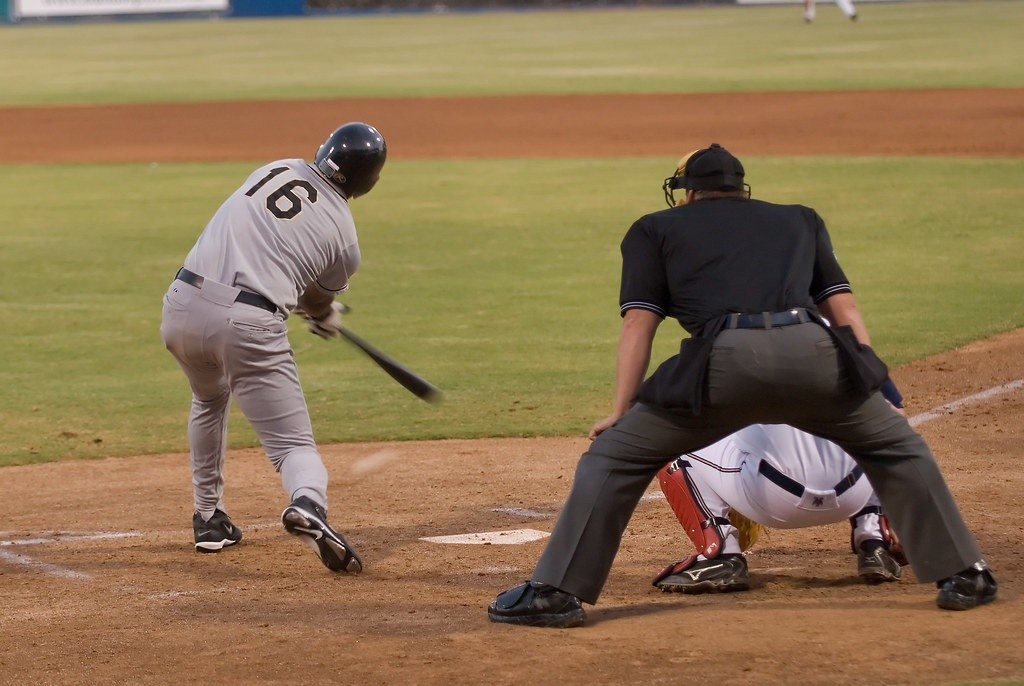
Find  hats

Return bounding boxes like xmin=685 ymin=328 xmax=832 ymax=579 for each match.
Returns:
xmin=669 ymin=143 xmax=745 ymax=190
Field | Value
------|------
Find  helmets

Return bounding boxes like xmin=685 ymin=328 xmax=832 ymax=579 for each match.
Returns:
xmin=315 ymin=123 xmax=387 ymax=200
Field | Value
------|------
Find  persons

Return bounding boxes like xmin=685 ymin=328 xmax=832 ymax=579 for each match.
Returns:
xmin=485 ymin=143 xmax=1000 ymax=627
xmin=159 ymin=122 xmax=388 ymax=576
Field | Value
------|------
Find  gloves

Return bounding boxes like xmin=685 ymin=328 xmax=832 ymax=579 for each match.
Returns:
xmin=291 ymin=299 xmax=349 ymax=341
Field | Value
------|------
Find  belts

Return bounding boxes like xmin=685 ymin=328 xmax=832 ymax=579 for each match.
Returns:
xmin=722 ymin=307 xmax=812 ymax=328
xmin=177 ymin=268 xmax=277 ymax=313
xmin=759 ymin=459 xmax=864 ymax=498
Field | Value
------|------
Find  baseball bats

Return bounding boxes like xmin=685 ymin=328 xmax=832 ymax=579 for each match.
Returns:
xmin=339 ymin=325 xmax=445 ymax=408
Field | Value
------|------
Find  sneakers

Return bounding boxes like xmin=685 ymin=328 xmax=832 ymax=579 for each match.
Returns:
xmin=488 ymin=579 xmax=585 ymax=628
xmin=657 ymin=553 xmax=749 ymax=594
xmin=193 ymin=509 xmax=243 ymax=556
xmin=281 ymin=495 xmax=362 ymax=577
xmin=858 ymin=541 xmax=903 ymax=581
xmin=937 ymin=558 xmax=998 ymax=610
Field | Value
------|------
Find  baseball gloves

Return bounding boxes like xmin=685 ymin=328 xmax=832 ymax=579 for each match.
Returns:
xmin=729 ymin=508 xmax=762 ymax=553
